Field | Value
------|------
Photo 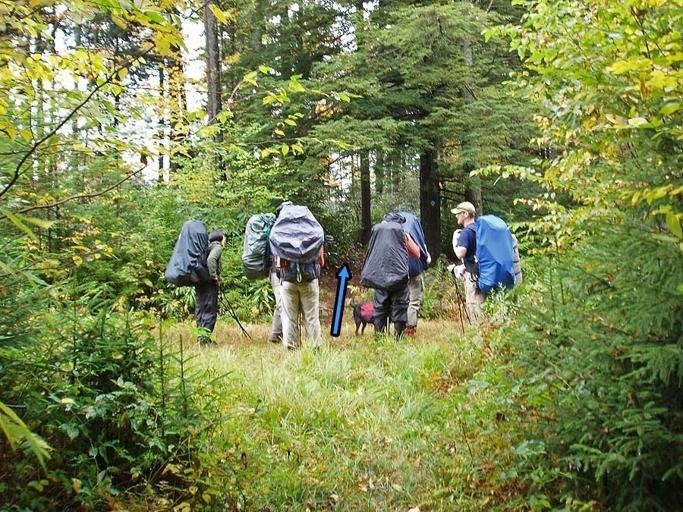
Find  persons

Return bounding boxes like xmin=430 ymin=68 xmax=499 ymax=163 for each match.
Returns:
xmin=446 ymin=259 xmax=468 ymax=282
xmin=373 ymin=232 xmax=421 ymax=343
xmin=193 ymin=230 xmax=226 ymax=346
xmin=450 ymin=201 xmax=488 ymax=344
xmin=268 ymin=201 xmax=294 ymax=344
xmin=405 ymin=273 xmax=431 ymax=338
xmin=273 ymin=250 xmax=325 ymax=352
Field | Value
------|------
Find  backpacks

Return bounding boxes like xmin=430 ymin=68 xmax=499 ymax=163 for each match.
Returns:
xmin=475 ymin=215 xmax=516 ymax=294
xmin=165 ymin=220 xmax=212 ymax=286
xmin=359 ymin=212 xmax=431 ymax=292
xmin=242 ymin=203 xmax=324 ymax=282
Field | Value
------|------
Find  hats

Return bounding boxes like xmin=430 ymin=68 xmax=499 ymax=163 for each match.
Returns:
xmin=450 ymin=201 xmax=476 ymax=214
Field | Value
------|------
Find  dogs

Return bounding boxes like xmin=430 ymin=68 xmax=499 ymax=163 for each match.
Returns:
xmin=349 ymin=299 xmax=392 ymax=336
